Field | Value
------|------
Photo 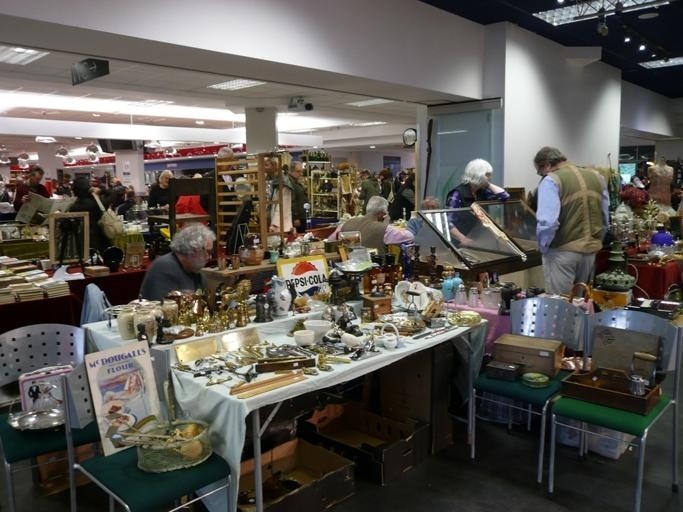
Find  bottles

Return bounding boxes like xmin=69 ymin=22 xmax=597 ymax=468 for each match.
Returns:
xmin=630 ymin=351 xmax=658 ymax=389
xmin=441 ymin=271 xmax=546 ymax=317
xmin=629 ymin=375 xmax=645 ymax=396
xmin=116 ymin=293 xmax=179 ymax=343
xmin=360 ymin=306 xmax=373 ymax=323
xmin=180 ymin=229 xmax=456 ymax=336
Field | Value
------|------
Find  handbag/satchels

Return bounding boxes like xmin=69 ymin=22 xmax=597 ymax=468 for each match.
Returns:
xmin=387 ymin=181 xmax=395 ymax=203
xmin=93 ymin=192 xmax=124 ymax=240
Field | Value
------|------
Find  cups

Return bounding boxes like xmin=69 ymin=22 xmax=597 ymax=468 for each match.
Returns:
xmin=373 ymin=333 xmax=397 ymax=350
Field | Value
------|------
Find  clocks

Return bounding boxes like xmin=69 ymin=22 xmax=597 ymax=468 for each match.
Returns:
xmin=403 ymin=128 xmax=416 ymax=146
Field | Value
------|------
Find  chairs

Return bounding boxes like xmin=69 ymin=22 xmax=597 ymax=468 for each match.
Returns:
xmin=469 ymin=297 xmax=583 ymax=483
xmin=148 ymin=178 xmax=216 ymax=241
xmin=0 ymin=320 xmax=114 ymax=511
xmin=549 ymin=310 xmax=683 ymax=512
xmin=61 ymin=348 xmax=247 ymax=512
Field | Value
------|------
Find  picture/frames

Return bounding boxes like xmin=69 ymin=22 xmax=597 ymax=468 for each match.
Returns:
xmin=49 ymin=212 xmax=90 ymax=266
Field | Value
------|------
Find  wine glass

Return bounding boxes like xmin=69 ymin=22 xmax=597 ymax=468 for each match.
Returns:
xmin=30 ymin=382 xmax=61 ymax=411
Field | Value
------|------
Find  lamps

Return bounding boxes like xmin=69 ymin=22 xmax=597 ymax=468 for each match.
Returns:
xmin=164 ymin=146 xmax=176 ymax=155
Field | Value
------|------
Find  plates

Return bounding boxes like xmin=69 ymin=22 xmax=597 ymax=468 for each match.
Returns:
xmin=109 ymin=413 xmax=136 ymax=432
xmin=7 ymin=408 xmax=65 ymax=431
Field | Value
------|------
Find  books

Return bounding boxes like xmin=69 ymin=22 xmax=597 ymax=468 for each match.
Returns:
xmin=82 ymin=336 xmax=167 ymax=458
xmin=0 ymin=250 xmax=72 ymax=307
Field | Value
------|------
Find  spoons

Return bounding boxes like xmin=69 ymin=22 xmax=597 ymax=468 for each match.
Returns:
xmin=168 ymin=340 xmax=318 ymax=380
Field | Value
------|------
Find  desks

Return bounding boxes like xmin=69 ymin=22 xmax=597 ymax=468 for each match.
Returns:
xmin=203 ymin=249 xmax=377 ymax=304
xmin=443 ymin=293 xmax=683 ymax=458
xmin=0 ymin=230 xmax=148 ymax=259
xmin=81 ymin=301 xmax=485 ymax=512
xmin=0 ymin=259 xmax=150 ymax=332
xmin=596 ymin=242 xmax=683 ymax=301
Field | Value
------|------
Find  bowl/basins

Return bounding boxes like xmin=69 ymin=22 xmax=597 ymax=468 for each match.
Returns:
xmin=102 ymin=401 xmax=125 ymax=417
xmin=519 ymin=371 xmax=550 ymax=388
xmin=302 ymin=319 xmax=332 ymax=338
xmin=293 ymin=329 xmax=315 ymax=347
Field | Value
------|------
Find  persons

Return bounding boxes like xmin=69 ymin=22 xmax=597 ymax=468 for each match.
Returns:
xmin=440 ymin=157 xmax=511 ymax=248
xmin=27 ymin=381 xmax=40 ymax=402
xmin=284 ymin=162 xmax=309 ymax=232
xmin=1 ymin=166 xmax=136 ymax=258
xmin=646 ymin=156 xmax=675 ymax=207
xmin=630 ymin=173 xmax=650 ymax=191
xmin=533 ymin=145 xmax=611 ymax=298
xmin=148 ymin=170 xmax=172 ymax=209
xmin=327 ymin=196 xmax=415 ymax=259
xmin=358 ymin=169 xmax=415 ymax=214
xmin=35 ymin=382 xmax=55 ymax=409
xmin=203 ymin=146 xmax=245 ymax=221
xmin=408 ymin=197 xmax=441 ymax=236
xmin=139 ymin=223 xmax=215 ymax=300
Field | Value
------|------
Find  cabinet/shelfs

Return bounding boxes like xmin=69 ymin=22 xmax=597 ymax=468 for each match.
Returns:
xmin=214 ymin=154 xmax=283 ymax=248
xmin=415 ymin=200 xmax=549 ymax=288
xmin=300 ymin=155 xmax=340 ymax=219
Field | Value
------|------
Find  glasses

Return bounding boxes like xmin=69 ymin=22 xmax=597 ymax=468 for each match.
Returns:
xmin=202 ymin=246 xmax=215 ymax=257
xmin=382 ymin=211 xmax=388 ymax=218
xmin=536 ymin=164 xmax=545 ymax=176
